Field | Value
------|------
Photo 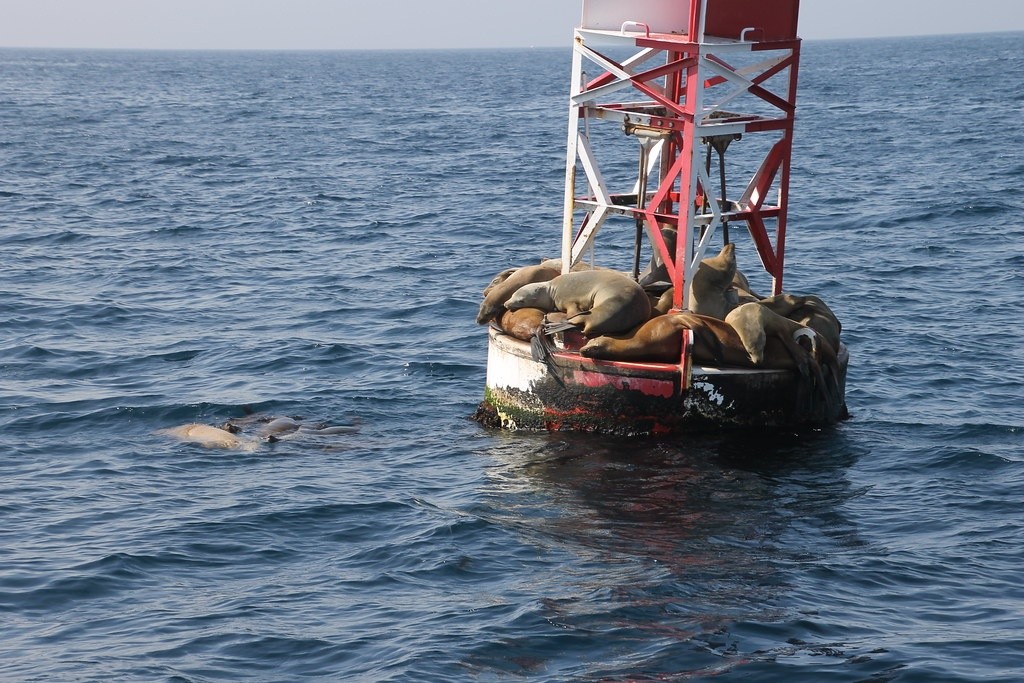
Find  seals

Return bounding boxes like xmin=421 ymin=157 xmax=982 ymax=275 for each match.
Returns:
xmin=162 ymin=412 xmax=362 ymax=455
xmin=475 ymin=210 xmax=842 ymax=414
